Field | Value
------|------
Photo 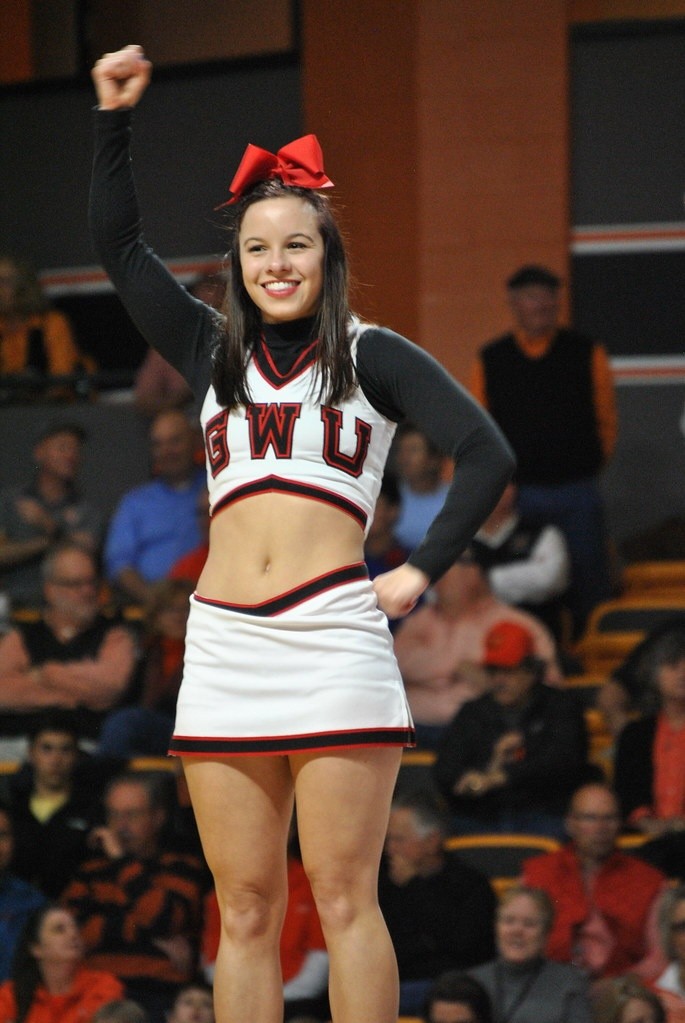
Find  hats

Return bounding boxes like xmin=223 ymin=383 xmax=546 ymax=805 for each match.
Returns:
xmin=476 ymin=620 xmax=535 ymax=669
xmin=505 ymin=263 xmax=559 ymax=289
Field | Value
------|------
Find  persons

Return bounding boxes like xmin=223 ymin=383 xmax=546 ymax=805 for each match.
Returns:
xmin=88 ymin=43 xmax=517 ymax=1023
xmin=473 ymin=886 xmax=596 ymax=1023
xmin=474 ymin=262 xmax=621 ymax=637
xmin=0 ymin=250 xmax=685 ymax=1023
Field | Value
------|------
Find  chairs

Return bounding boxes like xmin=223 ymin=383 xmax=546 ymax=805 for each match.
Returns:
xmin=0 ymin=558 xmax=685 ymax=904
